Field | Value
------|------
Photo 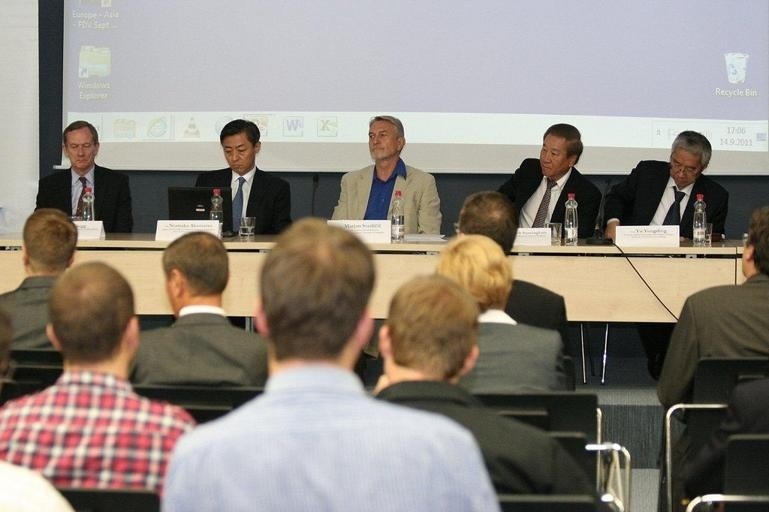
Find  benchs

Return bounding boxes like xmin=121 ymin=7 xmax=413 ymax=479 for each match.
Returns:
xmin=665 ymin=358 xmax=769 ymax=512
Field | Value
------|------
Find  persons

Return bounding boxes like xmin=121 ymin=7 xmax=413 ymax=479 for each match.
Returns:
xmin=332 ymin=115 xmax=442 ymax=363
xmin=130 ymin=232 xmax=267 ymax=386
xmin=0 ymin=211 xmax=197 ymax=512
xmin=681 ymin=380 xmax=769 ymax=512
xmin=369 ymin=192 xmax=599 ymax=512
xmin=499 ymin=123 xmax=600 ymax=238
xmin=162 ymin=217 xmax=500 ymax=512
xmin=187 ymin=120 xmax=292 ymax=235
xmin=34 ymin=121 xmax=133 ymax=232
xmin=656 ymin=207 xmax=769 ymax=422
xmin=603 ymin=131 xmax=729 ymax=380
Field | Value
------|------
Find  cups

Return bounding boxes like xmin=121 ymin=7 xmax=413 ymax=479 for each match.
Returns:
xmin=544 ymin=223 xmax=562 ymax=247
xmin=239 ymin=217 xmax=256 ymax=236
xmin=705 ymin=223 xmax=713 ymax=246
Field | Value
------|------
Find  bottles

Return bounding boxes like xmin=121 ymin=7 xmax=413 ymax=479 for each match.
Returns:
xmin=694 ymin=194 xmax=706 ymax=245
xmin=210 ymin=188 xmax=224 ymax=241
xmin=565 ymin=194 xmax=579 ymax=246
xmin=82 ymin=188 xmax=94 ymax=220
xmin=391 ymin=190 xmax=405 ymax=240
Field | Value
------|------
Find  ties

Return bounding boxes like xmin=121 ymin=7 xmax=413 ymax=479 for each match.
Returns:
xmin=231 ymin=176 xmax=246 ymax=234
xmin=662 ymin=185 xmax=685 ymax=225
xmin=531 ymin=177 xmax=559 ymax=228
xmin=75 ymin=176 xmax=86 ymax=217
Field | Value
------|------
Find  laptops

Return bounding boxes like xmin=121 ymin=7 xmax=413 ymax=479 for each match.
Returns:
xmin=168 ymin=186 xmax=238 ymax=237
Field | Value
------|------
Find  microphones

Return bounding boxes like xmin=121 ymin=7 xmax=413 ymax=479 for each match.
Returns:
xmin=310 ymin=172 xmax=319 ymax=215
xmin=587 ymin=177 xmax=614 ymax=245
